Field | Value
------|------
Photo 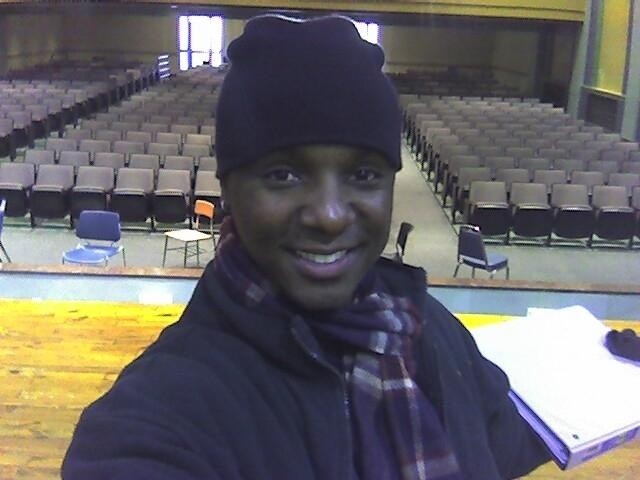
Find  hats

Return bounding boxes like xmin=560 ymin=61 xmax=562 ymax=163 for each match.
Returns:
xmin=215 ymin=15 xmax=403 ymax=178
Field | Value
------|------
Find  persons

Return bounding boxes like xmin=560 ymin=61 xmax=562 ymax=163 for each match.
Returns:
xmin=60 ymin=12 xmax=640 ymax=480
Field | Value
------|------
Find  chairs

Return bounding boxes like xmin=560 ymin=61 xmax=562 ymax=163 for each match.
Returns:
xmin=162 ymin=199 xmax=217 ymax=268
xmin=384 ymin=222 xmax=413 ymax=264
xmin=61 ymin=210 xmax=125 ymax=267
xmin=454 ymin=224 xmax=510 ymax=280
xmin=0 ymin=196 xmax=11 ymax=262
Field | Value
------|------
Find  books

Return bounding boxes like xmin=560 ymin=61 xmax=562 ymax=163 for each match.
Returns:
xmin=463 ymin=300 xmax=640 ymax=471
